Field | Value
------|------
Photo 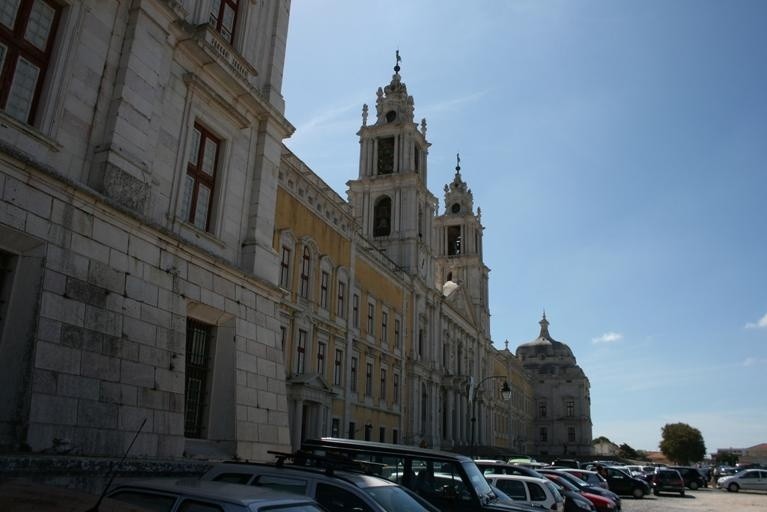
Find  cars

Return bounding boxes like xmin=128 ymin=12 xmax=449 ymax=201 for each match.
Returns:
xmin=106 ymin=478 xmax=328 ymax=512
xmin=716 ymin=462 xmax=767 ymax=492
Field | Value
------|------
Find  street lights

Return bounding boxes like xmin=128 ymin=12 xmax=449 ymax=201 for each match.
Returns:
xmin=471 ymin=376 xmax=512 ymax=458
